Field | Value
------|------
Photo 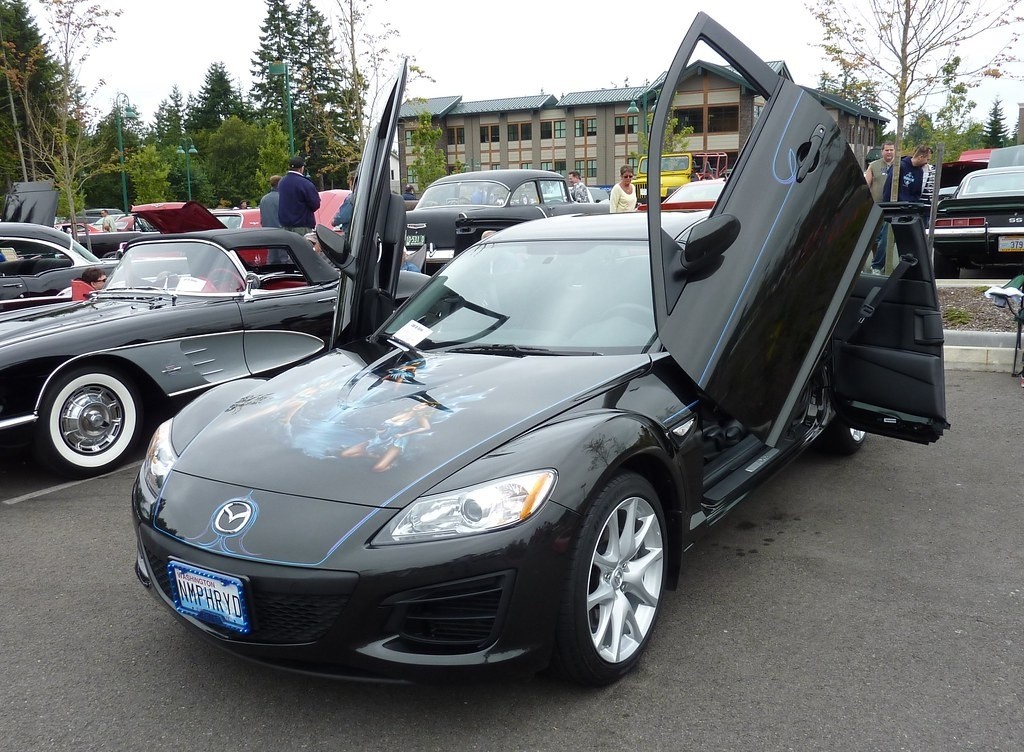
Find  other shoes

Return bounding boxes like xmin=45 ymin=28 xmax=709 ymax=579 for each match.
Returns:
xmin=870 ymin=266 xmax=882 ymax=276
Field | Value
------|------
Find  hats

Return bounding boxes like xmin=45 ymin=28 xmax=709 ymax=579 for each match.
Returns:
xmin=289 ymin=156 xmax=306 ymax=168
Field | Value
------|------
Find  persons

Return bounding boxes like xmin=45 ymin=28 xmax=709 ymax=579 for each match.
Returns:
xmin=329 ymin=170 xmax=359 ymax=242
xmin=240 ymin=200 xmax=247 ymax=209
xmin=402 ymin=184 xmax=416 ymax=199
xmin=400 ymin=245 xmax=420 ymax=272
xmin=567 ymin=171 xmax=594 ymax=203
xmin=259 ymin=175 xmax=284 ymax=228
xmin=481 ymin=230 xmax=496 ymax=242
xmin=609 ymin=164 xmax=637 ymax=213
xmin=278 ymin=156 xmax=321 ymax=238
xmin=864 ymin=141 xmax=938 ymax=275
xmin=100 ymin=209 xmax=117 ymax=232
xmin=81 ymin=266 xmax=107 ymax=290
xmin=541 ymin=170 xmax=557 ymax=195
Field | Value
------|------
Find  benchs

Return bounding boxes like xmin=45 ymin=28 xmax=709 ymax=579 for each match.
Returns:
xmin=264 ymin=282 xmax=306 ymax=290
xmin=0 ymin=259 xmax=73 ymax=276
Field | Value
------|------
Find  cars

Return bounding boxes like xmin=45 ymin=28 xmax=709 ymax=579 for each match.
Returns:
xmin=0 ymin=176 xmax=359 ymax=314
xmin=402 ymin=167 xmax=613 ymax=266
xmin=639 ymin=178 xmax=730 ymax=213
xmin=931 ymin=165 xmax=1024 ymax=280
xmin=0 ymin=226 xmax=434 ymax=479
xmin=128 ymin=10 xmax=953 ymax=689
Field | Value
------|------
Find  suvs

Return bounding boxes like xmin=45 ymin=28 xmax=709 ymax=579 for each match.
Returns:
xmin=630 ymin=150 xmax=730 ymax=201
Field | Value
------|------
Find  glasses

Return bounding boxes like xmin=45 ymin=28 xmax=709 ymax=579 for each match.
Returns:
xmin=96 ymin=279 xmax=106 ymax=282
xmin=622 ymin=175 xmax=633 ymax=178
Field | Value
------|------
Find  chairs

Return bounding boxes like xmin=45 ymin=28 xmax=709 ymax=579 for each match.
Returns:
xmin=989 ymin=275 xmax=1024 ymax=377
xmin=71 ymin=279 xmax=96 ymax=301
xmin=700 ymin=161 xmax=713 ymax=173
